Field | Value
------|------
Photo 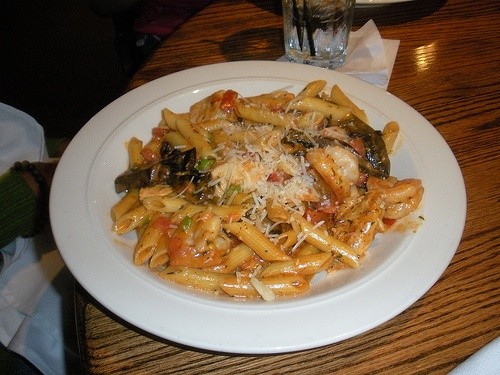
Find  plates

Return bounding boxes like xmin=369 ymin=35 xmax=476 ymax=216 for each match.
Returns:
xmin=49 ymin=61 xmax=465 ymax=355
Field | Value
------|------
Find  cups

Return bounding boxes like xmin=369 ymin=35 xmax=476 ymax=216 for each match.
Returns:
xmin=282 ymin=0 xmax=356 ymax=69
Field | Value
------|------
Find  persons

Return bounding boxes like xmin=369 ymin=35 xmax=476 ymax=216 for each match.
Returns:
xmin=0 ymin=159 xmax=57 ymax=256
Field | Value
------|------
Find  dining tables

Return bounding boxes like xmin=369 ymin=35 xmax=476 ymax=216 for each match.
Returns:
xmin=81 ymin=2 xmax=499 ymax=374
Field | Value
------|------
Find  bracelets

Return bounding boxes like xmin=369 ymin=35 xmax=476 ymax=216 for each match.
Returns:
xmin=11 ymin=161 xmax=48 ymax=237
